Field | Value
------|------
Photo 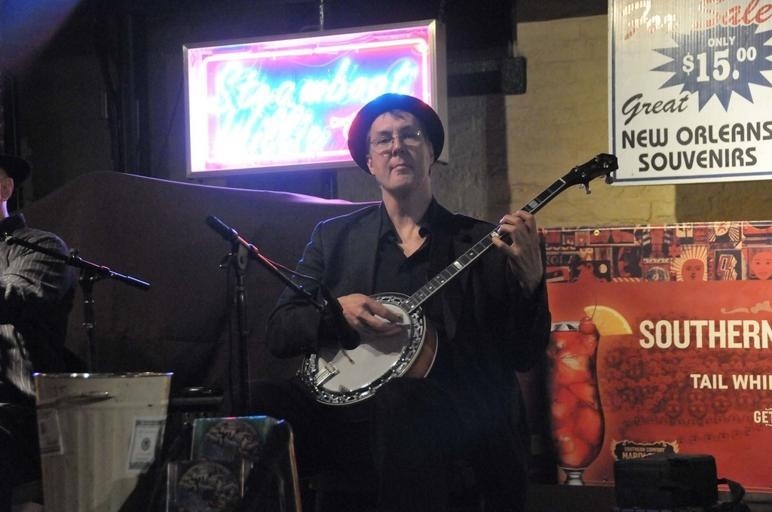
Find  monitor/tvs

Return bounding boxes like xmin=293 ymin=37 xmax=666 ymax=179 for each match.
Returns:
xmin=183 ymin=19 xmax=449 ymax=179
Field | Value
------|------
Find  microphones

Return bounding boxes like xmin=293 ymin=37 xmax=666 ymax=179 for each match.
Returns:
xmin=320 ymin=285 xmax=361 ymax=350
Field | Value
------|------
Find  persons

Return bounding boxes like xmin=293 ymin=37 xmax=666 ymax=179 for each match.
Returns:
xmin=614 ymin=222 xmax=772 ymax=280
xmin=265 ymin=91 xmax=550 ymax=512
xmin=1 ymin=135 xmax=76 ymax=512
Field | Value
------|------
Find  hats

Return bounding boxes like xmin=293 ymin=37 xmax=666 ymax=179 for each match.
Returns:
xmin=0 ymin=141 xmax=31 ymax=188
xmin=347 ymin=92 xmax=445 ymax=176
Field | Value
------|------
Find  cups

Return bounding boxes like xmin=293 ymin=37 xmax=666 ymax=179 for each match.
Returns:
xmin=547 ymin=320 xmax=602 ymax=485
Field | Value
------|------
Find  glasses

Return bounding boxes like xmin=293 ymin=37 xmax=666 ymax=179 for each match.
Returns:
xmin=366 ymin=127 xmax=427 ymax=155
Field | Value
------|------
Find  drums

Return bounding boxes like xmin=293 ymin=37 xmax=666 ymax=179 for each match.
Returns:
xmin=35 ymin=371 xmax=171 ymax=512
xmin=168 ymin=383 xmax=226 ymax=460
